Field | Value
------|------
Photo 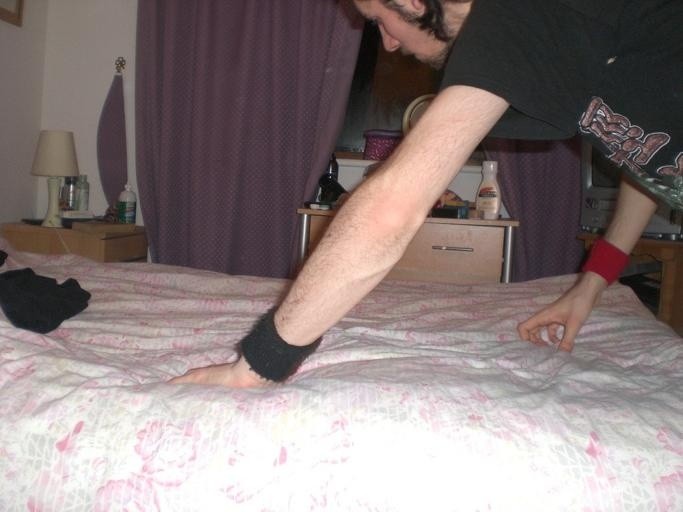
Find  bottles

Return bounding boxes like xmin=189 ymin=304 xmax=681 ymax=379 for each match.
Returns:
xmin=475 ymin=160 xmax=501 ymax=220
xmin=117 ymin=184 xmax=137 ymax=225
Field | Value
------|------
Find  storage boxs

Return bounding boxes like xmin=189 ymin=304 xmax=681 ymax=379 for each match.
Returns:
xmin=363 ymin=130 xmax=401 ymax=161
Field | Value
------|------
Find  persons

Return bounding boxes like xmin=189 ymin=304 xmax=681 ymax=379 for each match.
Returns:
xmin=166 ymin=1 xmax=683 ymax=389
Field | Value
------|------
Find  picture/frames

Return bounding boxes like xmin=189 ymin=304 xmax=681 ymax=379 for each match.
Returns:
xmin=0 ymin=1 xmax=24 ymax=28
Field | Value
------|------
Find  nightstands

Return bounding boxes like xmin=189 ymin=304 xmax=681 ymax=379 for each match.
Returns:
xmin=0 ymin=220 xmax=147 ymax=263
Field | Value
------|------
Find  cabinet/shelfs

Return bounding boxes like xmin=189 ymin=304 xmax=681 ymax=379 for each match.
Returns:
xmin=296 ymin=215 xmax=516 ymax=286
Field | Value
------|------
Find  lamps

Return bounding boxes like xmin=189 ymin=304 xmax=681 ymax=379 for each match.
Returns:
xmin=30 ymin=130 xmax=80 ymax=227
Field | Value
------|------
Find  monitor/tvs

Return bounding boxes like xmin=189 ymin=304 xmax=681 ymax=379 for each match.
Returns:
xmin=580 ymin=136 xmax=683 ymax=240
